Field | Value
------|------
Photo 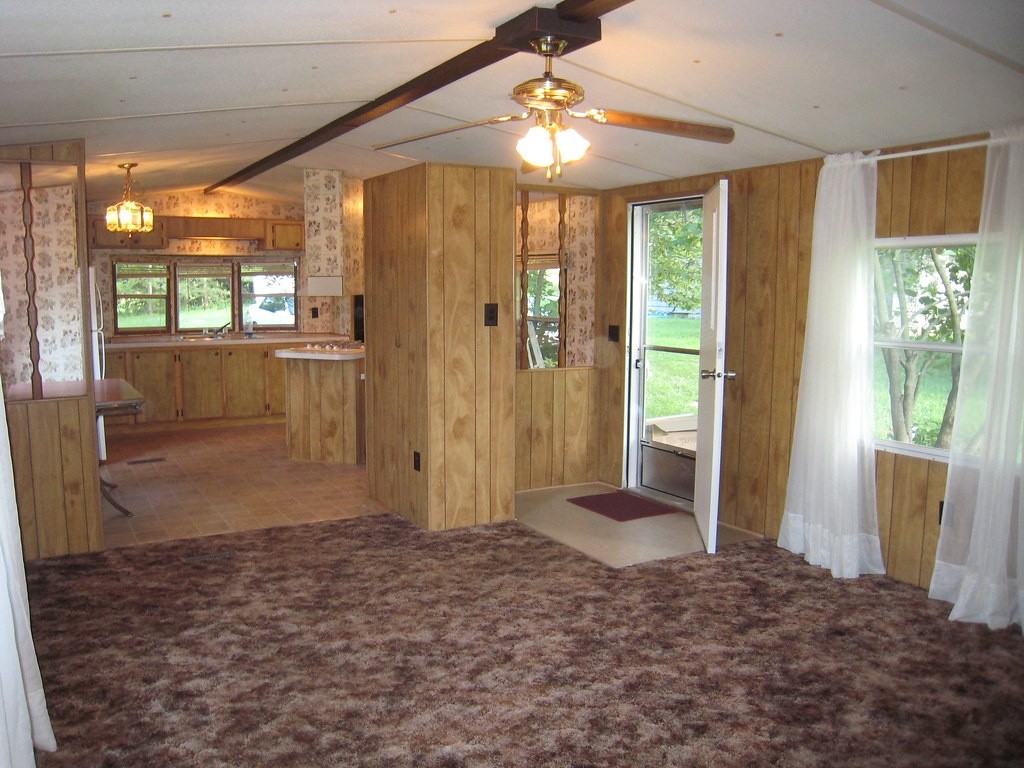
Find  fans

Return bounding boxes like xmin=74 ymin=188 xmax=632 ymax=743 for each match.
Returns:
xmin=373 ymin=6 xmax=734 ymax=175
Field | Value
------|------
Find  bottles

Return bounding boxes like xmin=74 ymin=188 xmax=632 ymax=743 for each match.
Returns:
xmin=243 ymin=311 xmax=253 ymax=332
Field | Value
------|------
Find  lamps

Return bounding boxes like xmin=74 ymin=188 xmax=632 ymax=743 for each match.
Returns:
xmin=512 ymin=104 xmax=592 ymax=167
xmin=106 ymin=164 xmax=153 ymax=239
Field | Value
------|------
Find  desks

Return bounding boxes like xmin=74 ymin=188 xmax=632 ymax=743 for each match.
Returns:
xmin=275 ymin=346 xmax=365 ymax=469
xmin=7 ymin=378 xmax=145 ymax=520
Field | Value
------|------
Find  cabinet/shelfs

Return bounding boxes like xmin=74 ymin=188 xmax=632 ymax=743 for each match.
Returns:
xmin=99 ymin=341 xmax=312 ymax=436
xmin=92 ymin=214 xmax=305 ymax=250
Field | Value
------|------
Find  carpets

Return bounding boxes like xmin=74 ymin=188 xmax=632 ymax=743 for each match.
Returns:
xmin=566 ymin=489 xmax=683 ymax=522
xmin=23 ymin=513 xmax=1024 ymax=768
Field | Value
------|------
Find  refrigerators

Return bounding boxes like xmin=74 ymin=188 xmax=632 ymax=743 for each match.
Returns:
xmin=88 ymin=264 xmax=108 ymax=463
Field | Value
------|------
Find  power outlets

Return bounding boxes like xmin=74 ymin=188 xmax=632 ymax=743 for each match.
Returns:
xmin=483 ymin=303 xmax=498 ymax=327
xmin=414 ymin=450 xmax=420 ymax=471
xmin=312 ymin=307 xmax=319 ymax=319
